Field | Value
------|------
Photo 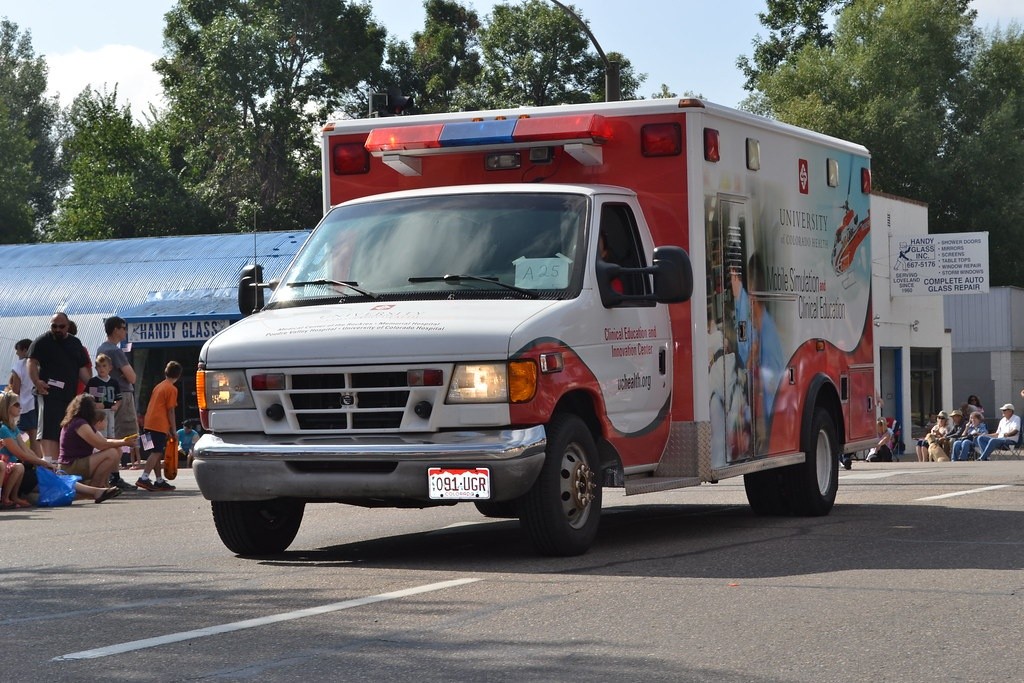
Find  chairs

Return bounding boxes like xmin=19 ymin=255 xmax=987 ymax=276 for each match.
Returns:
xmin=996 ymin=427 xmax=1022 ymax=460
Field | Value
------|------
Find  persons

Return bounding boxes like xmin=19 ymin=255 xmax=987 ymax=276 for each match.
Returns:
xmin=135 ymin=360 xmax=182 ymax=491
xmin=82 ymin=317 xmax=137 ymax=493
xmin=707 ymin=238 xmax=785 ymax=463
xmin=598 ymin=236 xmax=624 ymax=296
xmin=178 ymin=419 xmax=198 ymax=468
xmin=913 ymin=410 xmax=991 ymax=461
xmin=54 ymin=394 xmax=139 ymax=494
xmin=868 ymin=417 xmax=893 ymax=462
xmin=966 ymin=395 xmax=985 ymax=417
xmin=977 ymin=403 xmax=1021 ymax=461
xmin=0 ymin=392 xmax=56 ymax=510
xmin=10 ymin=312 xmax=93 ymax=468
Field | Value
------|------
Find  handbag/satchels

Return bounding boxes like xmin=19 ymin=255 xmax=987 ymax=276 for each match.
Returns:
xmin=35 ymin=466 xmax=83 ymax=507
xmin=164 ymin=437 xmax=179 ymax=481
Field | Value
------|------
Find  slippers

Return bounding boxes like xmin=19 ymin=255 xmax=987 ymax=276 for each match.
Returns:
xmin=94 ymin=487 xmax=122 ymax=504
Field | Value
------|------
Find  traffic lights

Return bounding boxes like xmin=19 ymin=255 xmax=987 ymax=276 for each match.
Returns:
xmin=374 ymin=88 xmax=416 ymax=115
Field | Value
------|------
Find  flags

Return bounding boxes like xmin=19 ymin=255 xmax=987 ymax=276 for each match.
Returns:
xmin=138 ymin=432 xmax=154 ymax=450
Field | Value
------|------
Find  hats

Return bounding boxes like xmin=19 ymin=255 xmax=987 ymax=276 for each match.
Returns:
xmin=183 ymin=419 xmax=193 ymax=428
xmin=948 ymin=410 xmax=964 ymax=418
xmin=1000 ymin=404 xmax=1014 ymax=411
xmin=936 ymin=411 xmax=949 ymax=420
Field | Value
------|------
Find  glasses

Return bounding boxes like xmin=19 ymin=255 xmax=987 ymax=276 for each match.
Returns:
xmin=120 ymin=327 xmax=127 ymax=331
xmin=938 ymin=416 xmax=945 ymax=420
xmin=1002 ymin=409 xmax=1010 ymax=411
xmin=13 ymin=402 xmax=20 ymax=408
xmin=970 ymin=399 xmax=977 ymax=401
xmin=51 ymin=323 xmax=67 ymax=329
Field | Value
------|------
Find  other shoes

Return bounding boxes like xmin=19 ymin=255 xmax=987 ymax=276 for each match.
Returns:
xmin=110 ymin=478 xmax=138 ymax=491
xmin=0 ymin=498 xmax=27 ymax=509
xmin=843 ymin=458 xmax=852 ymax=470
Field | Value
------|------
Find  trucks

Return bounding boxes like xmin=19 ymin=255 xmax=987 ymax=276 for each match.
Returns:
xmin=192 ymin=95 xmax=880 ymax=553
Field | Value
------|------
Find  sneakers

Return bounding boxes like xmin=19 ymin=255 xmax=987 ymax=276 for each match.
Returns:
xmin=154 ymin=479 xmax=176 ymax=491
xmin=135 ymin=477 xmax=158 ymax=491
